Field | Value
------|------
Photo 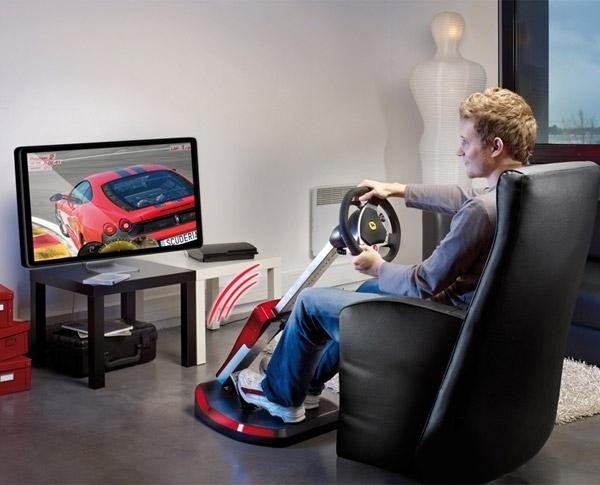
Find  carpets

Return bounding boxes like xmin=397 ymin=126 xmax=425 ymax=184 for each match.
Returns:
xmin=557 ymin=356 xmax=600 ymax=432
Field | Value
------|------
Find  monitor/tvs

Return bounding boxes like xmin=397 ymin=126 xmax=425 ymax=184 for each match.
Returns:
xmin=13 ymin=136 xmax=204 ymax=270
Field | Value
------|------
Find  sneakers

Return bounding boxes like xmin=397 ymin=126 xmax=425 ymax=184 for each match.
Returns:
xmin=259 ymin=357 xmax=320 ymax=409
xmin=238 ymin=369 xmax=306 ymax=423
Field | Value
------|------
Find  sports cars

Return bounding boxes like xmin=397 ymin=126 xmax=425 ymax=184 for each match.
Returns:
xmin=50 ymin=162 xmax=200 ymax=254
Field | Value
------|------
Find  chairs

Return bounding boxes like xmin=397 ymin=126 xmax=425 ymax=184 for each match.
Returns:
xmin=333 ymin=159 xmax=595 ymax=475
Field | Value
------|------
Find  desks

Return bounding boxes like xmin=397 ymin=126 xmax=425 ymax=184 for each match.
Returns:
xmin=23 ymin=255 xmax=280 ymax=394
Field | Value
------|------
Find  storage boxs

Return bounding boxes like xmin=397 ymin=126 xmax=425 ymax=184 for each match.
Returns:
xmin=0 ymin=284 xmax=33 ymax=397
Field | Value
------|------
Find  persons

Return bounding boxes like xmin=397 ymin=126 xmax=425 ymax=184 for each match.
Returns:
xmin=236 ymin=90 xmax=539 ymax=426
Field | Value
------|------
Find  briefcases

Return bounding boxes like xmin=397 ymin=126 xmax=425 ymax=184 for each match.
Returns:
xmin=44 ymin=318 xmax=156 ymax=378
